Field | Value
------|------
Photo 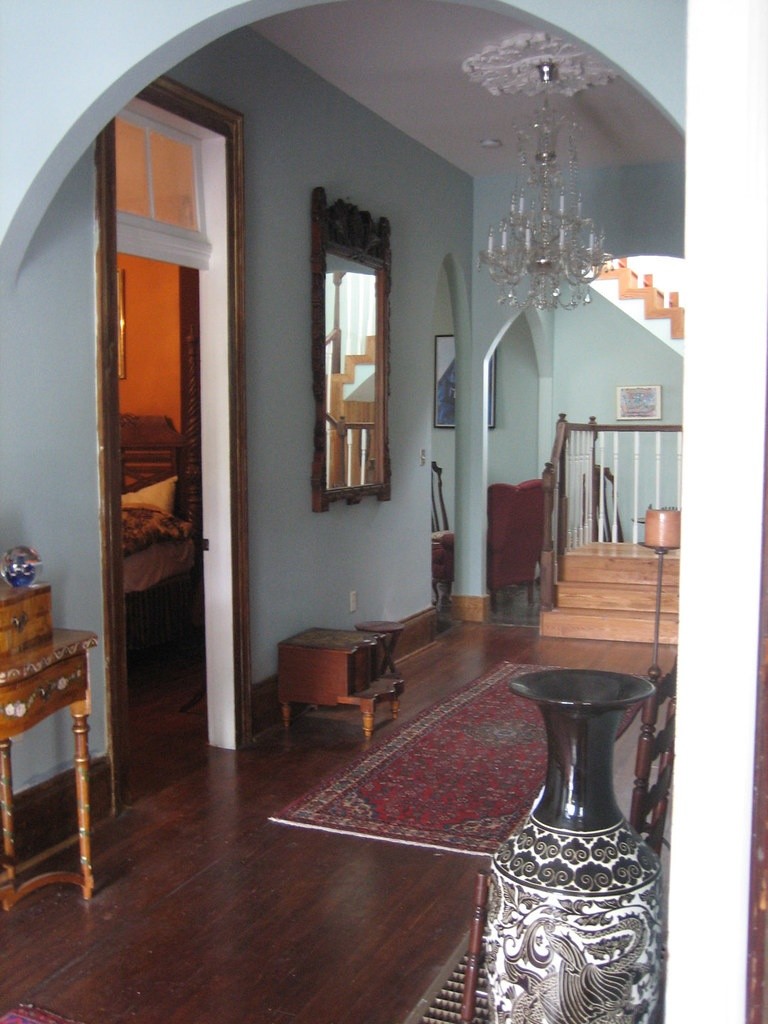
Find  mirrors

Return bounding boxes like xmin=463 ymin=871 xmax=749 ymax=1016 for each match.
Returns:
xmin=310 ymin=185 xmax=392 ymax=513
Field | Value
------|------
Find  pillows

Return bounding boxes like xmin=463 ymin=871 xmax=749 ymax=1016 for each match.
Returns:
xmin=121 ymin=475 xmax=178 ymax=513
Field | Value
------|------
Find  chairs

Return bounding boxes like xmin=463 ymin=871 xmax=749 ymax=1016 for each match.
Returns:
xmin=462 ymin=657 xmax=676 ymax=1023
xmin=432 ymin=478 xmax=544 ymax=608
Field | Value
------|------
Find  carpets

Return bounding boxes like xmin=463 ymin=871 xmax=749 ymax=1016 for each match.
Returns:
xmin=0 ymin=1002 xmax=88 ymax=1024
xmin=267 ymin=661 xmax=645 ymax=857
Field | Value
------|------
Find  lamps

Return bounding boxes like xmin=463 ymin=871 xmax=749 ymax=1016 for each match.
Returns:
xmin=638 ymin=507 xmax=680 ymax=665
xmin=476 ymin=60 xmax=614 ymax=313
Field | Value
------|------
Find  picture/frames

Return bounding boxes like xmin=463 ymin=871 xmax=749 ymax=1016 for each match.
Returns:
xmin=434 ymin=334 xmax=495 ymax=429
xmin=616 ymin=385 xmax=662 ymax=421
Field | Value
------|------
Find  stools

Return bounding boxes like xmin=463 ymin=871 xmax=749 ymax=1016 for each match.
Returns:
xmin=356 ymin=620 xmax=404 ymax=678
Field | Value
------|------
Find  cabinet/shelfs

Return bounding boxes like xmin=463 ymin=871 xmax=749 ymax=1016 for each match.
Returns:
xmin=277 ymin=627 xmax=405 ymax=742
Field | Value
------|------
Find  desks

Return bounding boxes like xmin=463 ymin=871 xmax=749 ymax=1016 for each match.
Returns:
xmin=0 ymin=626 xmax=99 ymax=912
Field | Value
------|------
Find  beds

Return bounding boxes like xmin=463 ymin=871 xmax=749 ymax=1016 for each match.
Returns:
xmin=118 ymin=413 xmax=203 ymax=662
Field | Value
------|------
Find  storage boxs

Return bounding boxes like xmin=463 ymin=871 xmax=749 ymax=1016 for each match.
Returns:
xmin=1 ymin=579 xmax=53 ymax=662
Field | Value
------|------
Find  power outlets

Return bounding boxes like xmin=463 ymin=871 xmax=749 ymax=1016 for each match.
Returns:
xmin=350 ymin=591 xmax=357 ymax=611
